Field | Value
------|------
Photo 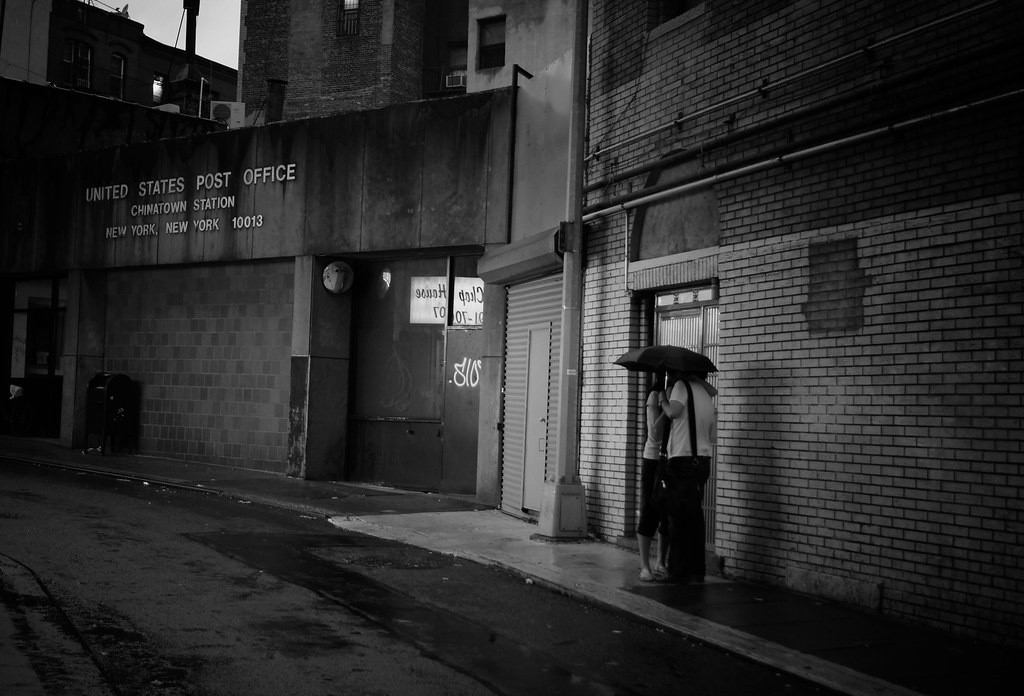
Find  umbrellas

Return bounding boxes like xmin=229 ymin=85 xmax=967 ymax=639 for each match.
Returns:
xmin=613 ymin=345 xmax=719 ymax=374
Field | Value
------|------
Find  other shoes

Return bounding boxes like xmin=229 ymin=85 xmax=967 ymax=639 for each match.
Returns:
xmin=664 ymin=576 xmax=689 ymax=584
xmin=691 ymin=574 xmax=704 ymax=583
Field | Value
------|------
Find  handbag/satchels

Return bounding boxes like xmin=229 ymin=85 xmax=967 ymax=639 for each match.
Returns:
xmin=644 ymin=462 xmax=702 ymax=517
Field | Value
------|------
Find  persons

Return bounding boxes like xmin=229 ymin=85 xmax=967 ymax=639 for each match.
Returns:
xmin=659 ymin=373 xmax=717 ymax=586
xmin=637 ymin=374 xmax=679 ymax=581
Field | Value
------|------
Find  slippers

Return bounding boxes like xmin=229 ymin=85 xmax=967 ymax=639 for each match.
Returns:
xmin=637 ymin=567 xmax=654 ymax=582
xmin=651 ymin=566 xmax=673 ymax=582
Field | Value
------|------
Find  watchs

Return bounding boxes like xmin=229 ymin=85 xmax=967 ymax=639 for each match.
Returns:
xmin=658 ymin=399 xmax=668 ymax=407
